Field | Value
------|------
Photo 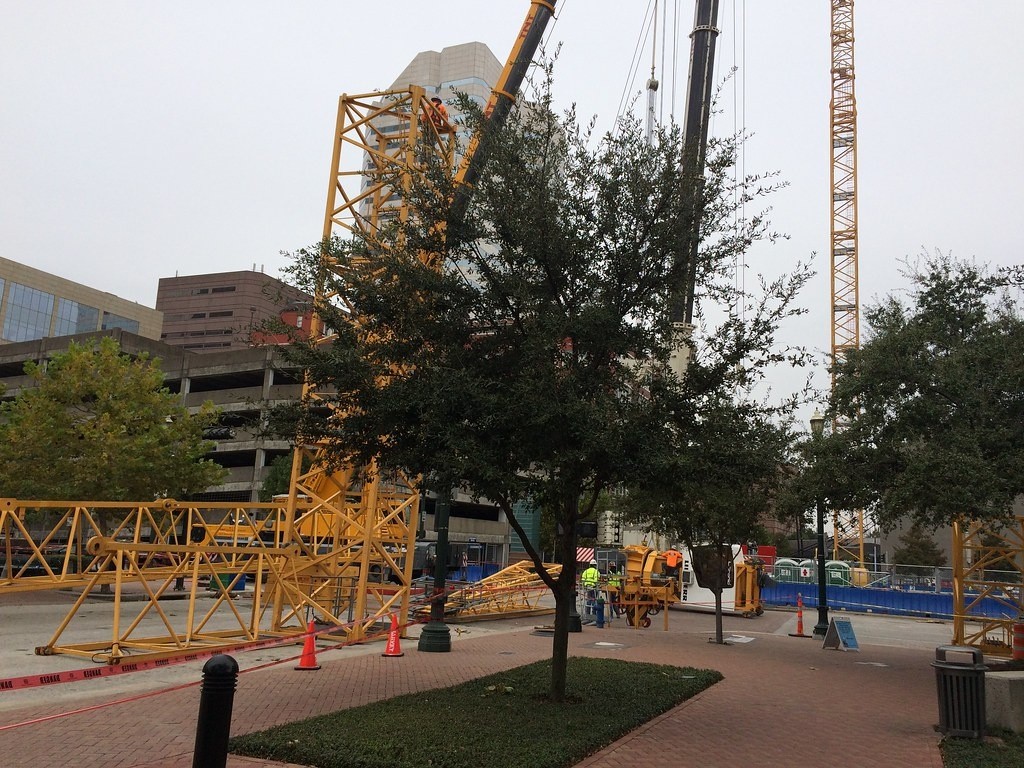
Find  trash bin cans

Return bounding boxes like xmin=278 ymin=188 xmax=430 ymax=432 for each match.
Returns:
xmin=934 ymin=644 xmax=988 ymax=744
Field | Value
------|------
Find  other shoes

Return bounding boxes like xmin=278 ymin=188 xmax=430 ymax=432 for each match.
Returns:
xmin=617 ymin=613 xmax=620 ymax=618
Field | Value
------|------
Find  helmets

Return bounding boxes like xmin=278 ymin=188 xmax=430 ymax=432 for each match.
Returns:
xmin=609 ymin=562 xmax=616 ymax=566
xmin=590 ymin=559 xmax=597 ymax=564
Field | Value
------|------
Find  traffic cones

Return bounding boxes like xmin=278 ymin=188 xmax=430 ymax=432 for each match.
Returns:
xmin=381 ymin=613 xmax=404 ymax=657
xmin=294 ymin=620 xmax=322 ymax=670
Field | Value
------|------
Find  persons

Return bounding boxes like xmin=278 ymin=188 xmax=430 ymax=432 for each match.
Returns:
xmin=604 ymin=561 xmax=621 ymax=618
xmin=421 ymin=96 xmax=448 ymax=145
xmin=626 ymin=576 xmax=653 ymax=618
xmin=662 ymin=544 xmax=682 ymax=577
xmin=461 ymin=552 xmax=468 ymax=577
xmin=582 ymin=559 xmax=600 ymax=615
xmin=751 ymin=564 xmax=765 ymax=604
